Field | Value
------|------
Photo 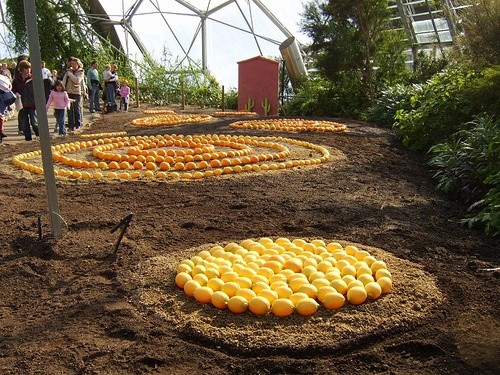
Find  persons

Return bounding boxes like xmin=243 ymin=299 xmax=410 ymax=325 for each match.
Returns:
xmin=102 ymin=64 xmax=119 ymax=112
xmin=41 ymin=57 xmax=87 ymax=132
xmin=87 ymin=61 xmax=102 ymax=113
xmin=0 ymin=55 xmax=40 ymax=145
xmin=118 ymin=79 xmax=131 ymax=113
xmin=46 ymin=81 xmax=70 ymax=136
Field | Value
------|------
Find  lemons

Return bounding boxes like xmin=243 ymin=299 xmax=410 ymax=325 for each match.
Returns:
xmin=176 ymin=236 xmax=392 ymax=316
xmin=229 ymin=119 xmax=347 ymax=132
xmin=12 ymin=130 xmax=330 ymax=179
xmin=132 ymin=110 xmax=256 ymax=126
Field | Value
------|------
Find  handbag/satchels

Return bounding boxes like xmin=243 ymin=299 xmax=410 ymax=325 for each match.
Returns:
xmin=0 ymin=90 xmax=17 ymax=106
